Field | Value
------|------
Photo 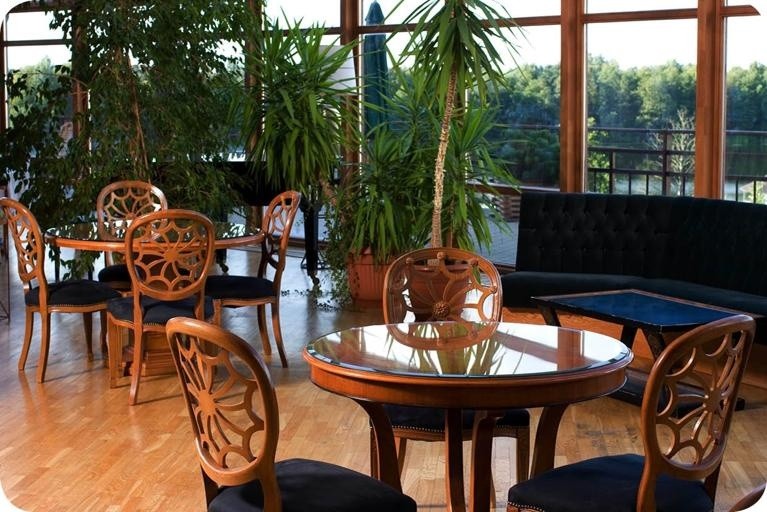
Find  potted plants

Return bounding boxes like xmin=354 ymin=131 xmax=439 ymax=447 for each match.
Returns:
xmin=344 ymin=122 xmax=412 ymax=311
xmin=395 ymin=0 xmax=522 ymax=321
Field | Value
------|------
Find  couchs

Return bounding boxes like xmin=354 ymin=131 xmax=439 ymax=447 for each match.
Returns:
xmin=500 ymin=190 xmax=766 ymax=309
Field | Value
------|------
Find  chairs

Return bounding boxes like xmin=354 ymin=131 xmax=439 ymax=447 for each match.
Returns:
xmin=204 ymin=190 xmax=303 ymax=368
xmin=96 ymin=180 xmax=169 ymax=289
xmin=1 ymin=197 xmax=122 ymax=384
xmin=107 ymin=209 xmax=215 ymax=407
xmin=369 ymin=247 xmax=529 ymax=512
xmin=509 ymin=314 xmax=757 ymax=512
xmin=164 ymin=316 xmax=417 ymax=512
